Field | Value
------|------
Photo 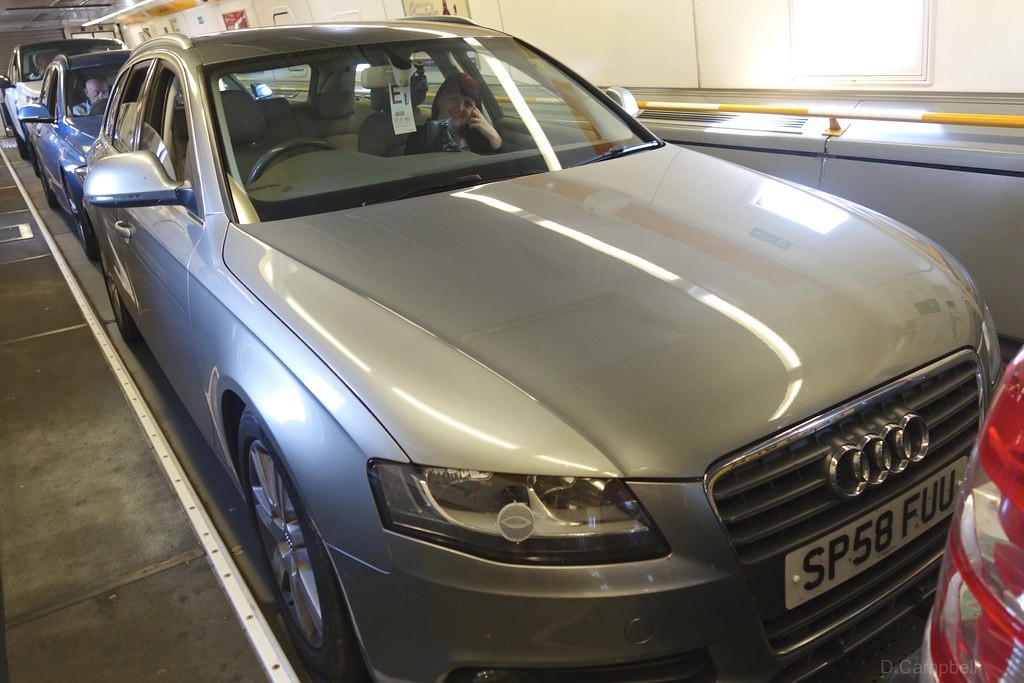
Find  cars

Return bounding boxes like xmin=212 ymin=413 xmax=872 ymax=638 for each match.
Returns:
xmin=15 ymin=48 xmax=273 ymax=264
xmin=0 ymin=38 xmax=129 ymax=161
xmin=85 ymin=13 xmax=1003 ymax=682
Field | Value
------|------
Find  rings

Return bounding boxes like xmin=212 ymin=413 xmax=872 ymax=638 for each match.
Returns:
xmin=475 ymin=117 xmax=478 ymax=122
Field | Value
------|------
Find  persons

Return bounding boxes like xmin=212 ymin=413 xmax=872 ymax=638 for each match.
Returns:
xmin=405 ymin=74 xmax=510 ymax=155
xmin=72 ymin=75 xmax=110 ymax=116
xmin=26 ymin=54 xmax=54 ymax=80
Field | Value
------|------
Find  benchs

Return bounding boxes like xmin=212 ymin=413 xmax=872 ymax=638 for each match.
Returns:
xmin=256 ymin=88 xmax=373 ymax=158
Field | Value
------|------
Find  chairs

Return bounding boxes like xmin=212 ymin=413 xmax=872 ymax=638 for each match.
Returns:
xmin=184 ymin=89 xmax=270 ymax=190
xmin=357 ymin=76 xmax=434 ymax=157
